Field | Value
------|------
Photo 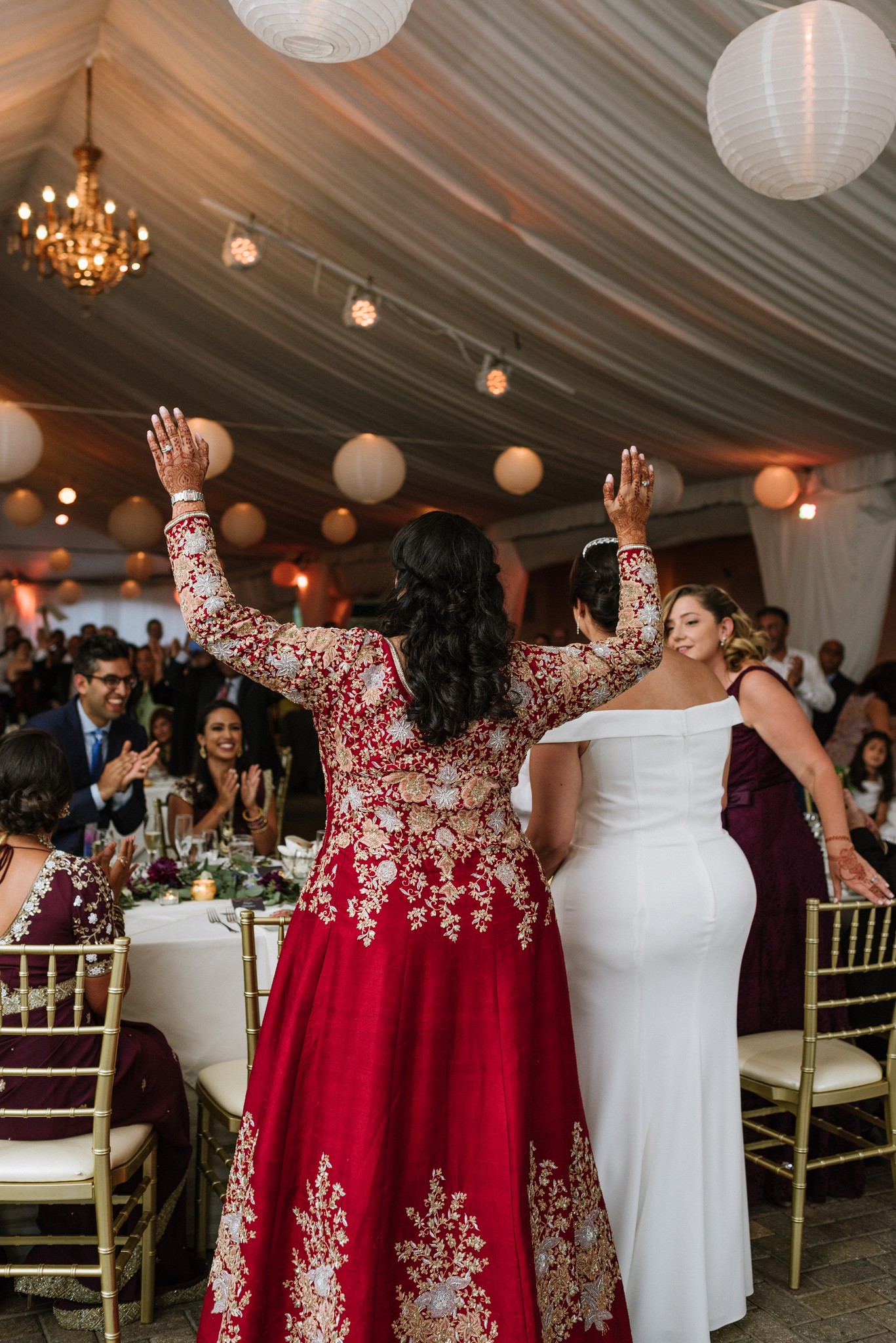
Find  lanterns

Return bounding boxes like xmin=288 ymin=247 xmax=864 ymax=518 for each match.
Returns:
xmin=220 ymin=503 xmax=268 ymax=550
xmin=701 ymin=1 xmax=896 ymax=203
xmin=172 ymin=585 xmax=179 ymax=604
xmin=169 ymin=417 xmax=236 ymax=482
xmin=636 ymin=455 xmax=684 ymax=515
xmin=331 ymin=433 xmax=408 ymax=506
xmin=1 ymin=489 xmax=45 ymax=528
xmin=47 ymin=548 xmax=72 ymax=572
xmin=56 ymin=579 xmax=82 ymax=604
xmin=226 ymin=2 xmax=413 ymax=64
xmin=124 ymin=549 xmax=153 ymax=582
xmin=752 ymin=464 xmax=802 ymax=510
xmin=106 ymin=497 xmax=165 ymax=551
xmin=494 ymin=445 xmax=545 ymax=496
xmin=0 ymin=398 xmax=46 ymax=484
xmin=270 ymin=561 xmax=303 ymax=589
xmin=120 ymin=581 xmax=140 ymax=597
xmin=1 ymin=578 xmax=18 ymax=604
xmin=319 ymin=509 xmax=358 ymax=546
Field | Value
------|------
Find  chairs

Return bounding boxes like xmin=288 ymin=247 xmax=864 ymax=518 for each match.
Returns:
xmin=153 ymin=796 xmax=195 ymax=859
xmin=268 ymin=744 xmax=297 ymax=862
xmin=733 ymin=897 xmax=896 ymax=1293
xmin=0 ymin=935 xmax=165 ymax=1343
xmin=187 ymin=909 xmax=292 ymax=1257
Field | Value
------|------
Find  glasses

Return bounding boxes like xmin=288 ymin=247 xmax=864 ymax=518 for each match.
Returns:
xmin=81 ymin=672 xmax=138 ymax=690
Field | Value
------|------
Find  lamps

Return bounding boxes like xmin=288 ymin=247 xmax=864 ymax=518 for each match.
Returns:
xmin=0 ymin=55 xmax=155 ymax=317
xmin=340 ymin=284 xmax=383 ymax=329
xmin=219 ymin=220 xmax=259 ymax=270
xmin=477 ymin=352 xmax=516 ymax=397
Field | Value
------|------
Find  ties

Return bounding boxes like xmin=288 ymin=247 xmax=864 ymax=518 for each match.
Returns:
xmin=84 ymin=730 xmax=104 ymax=858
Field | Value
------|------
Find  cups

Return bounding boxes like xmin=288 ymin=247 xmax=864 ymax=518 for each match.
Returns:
xmin=159 ymin=889 xmax=180 ymax=905
xmin=191 ymin=879 xmax=216 ymax=900
xmin=292 ymin=848 xmax=314 ymax=885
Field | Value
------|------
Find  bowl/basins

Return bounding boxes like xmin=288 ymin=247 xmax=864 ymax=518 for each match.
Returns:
xmin=277 ymin=836 xmax=324 ymax=875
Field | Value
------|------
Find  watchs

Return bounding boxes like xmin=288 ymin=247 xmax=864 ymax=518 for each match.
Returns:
xmin=169 ymin=489 xmax=205 ymax=507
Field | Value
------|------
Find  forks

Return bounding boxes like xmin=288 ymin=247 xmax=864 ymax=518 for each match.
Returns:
xmin=206 ymin=907 xmax=239 ymax=933
xmin=224 ymin=907 xmax=242 ymax=930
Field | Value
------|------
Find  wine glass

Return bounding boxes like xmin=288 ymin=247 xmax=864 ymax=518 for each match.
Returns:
xmin=91 ymin=813 xmax=254 ymax=873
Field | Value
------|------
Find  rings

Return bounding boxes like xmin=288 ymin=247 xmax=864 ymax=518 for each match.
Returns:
xmin=160 ymin=445 xmax=173 ymax=454
xmin=640 ymin=479 xmax=650 ymax=487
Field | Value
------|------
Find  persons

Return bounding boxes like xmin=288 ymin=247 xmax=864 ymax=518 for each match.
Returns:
xmin=145 ymin=404 xmax=665 ymax=1343
xmin=525 ymin=542 xmax=761 ymax=1343
xmin=0 ymin=732 xmax=214 ymax=1293
xmin=75 ymin=512 xmax=894 ymax=1057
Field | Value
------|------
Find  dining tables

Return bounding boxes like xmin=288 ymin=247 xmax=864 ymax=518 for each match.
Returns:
xmin=141 ymin=770 xmax=197 ymax=853
xmin=112 ymin=859 xmax=303 ymax=1245
